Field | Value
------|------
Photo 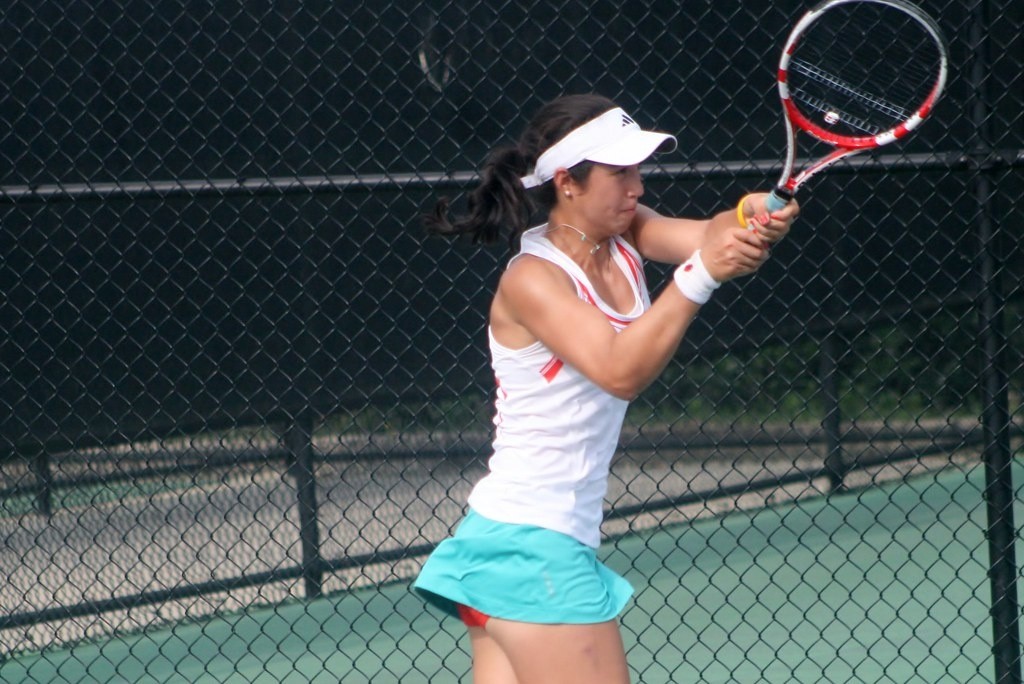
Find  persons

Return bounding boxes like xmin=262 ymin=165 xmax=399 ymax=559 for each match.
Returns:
xmin=410 ymin=94 xmax=803 ymax=684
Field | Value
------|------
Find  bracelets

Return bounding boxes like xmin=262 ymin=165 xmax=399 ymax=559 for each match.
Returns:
xmin=737 ymin=192 xmax=762 ymax=228
xmin=672 ymin=246 xmax=721 ymax=305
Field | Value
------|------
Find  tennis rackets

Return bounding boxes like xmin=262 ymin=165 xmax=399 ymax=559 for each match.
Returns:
xmin=747 ymin=1 xmax=952 ymax=234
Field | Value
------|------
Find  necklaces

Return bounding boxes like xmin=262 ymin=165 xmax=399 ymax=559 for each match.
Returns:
xmin=544 ymin=220 xmax=612 ymax=253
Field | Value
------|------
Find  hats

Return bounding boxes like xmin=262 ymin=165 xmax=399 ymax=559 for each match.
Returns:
xmin=520 ymin=107 xmax=677 ymax=190
